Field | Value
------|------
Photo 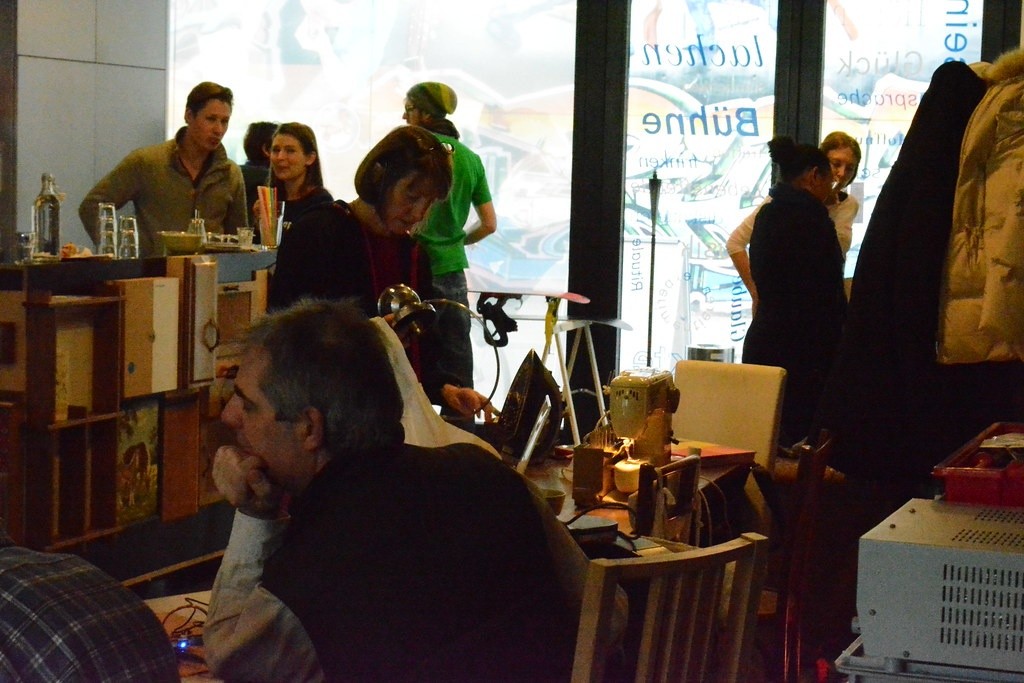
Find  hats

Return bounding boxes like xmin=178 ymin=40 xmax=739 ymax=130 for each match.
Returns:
xmin=408 ymin=82 xmax=458 ymax=120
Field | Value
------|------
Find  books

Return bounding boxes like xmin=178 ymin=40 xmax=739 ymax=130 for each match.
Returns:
xmin=671 ymin=437 xmax=756 ymax=468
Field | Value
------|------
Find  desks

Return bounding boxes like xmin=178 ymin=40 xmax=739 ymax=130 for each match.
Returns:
xmin=508 ymin=428 xmax=758 ymax=548
xmin=141 ymin=584 xmax=236 ymax=683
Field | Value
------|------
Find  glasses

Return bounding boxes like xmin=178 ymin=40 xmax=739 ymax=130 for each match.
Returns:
xmin=385 ymin=139 xmax=456 ymax=174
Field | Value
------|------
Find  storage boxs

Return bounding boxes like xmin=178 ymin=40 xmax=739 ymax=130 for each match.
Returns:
xmin=931 ymin=422 xmax=1024 ymax=508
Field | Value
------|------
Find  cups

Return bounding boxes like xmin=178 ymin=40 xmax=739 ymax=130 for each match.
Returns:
xmin=235 ymin=226 xmax=254 ymax=247
xmin=97 ymin=201 xmax=139 ymax=259
xmin=208 ymin=233 xmax=237 ymax=246
xmin=258 ymin=201 xmax=286 ymax=249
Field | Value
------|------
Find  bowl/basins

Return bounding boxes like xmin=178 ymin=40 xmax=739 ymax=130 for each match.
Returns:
xmin=540 ymin=488 xmax=564 ymax=516
xmin=158 ymin=230 xmax=205 ymax=255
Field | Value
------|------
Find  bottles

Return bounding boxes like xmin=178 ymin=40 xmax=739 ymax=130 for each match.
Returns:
xmin=188 ymin=218 xmax=205 ymax=255
xmin=30 ymin=173 xmax=61 ymax=257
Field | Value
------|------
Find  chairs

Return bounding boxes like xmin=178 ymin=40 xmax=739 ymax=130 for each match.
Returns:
xmin=670 ymin=360 xmax=788 ymax=480
xmin=570 ymin=531 xmax=769 ymax=682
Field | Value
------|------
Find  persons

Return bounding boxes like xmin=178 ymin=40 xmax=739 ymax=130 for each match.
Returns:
xmin=77 ymin=76 xmax=252 ymax=261
xmin=725 ymin=129 xmax=862 ymax=320
xmin=746 ymin=135 xmax=855 ymax=441
xmin=236 ymin=120 xmax=283 ymax=245
xmin=198 ymin=297 xmax=626 ymax=683
xmin=401 ymin=79 xmax=496 ymax=389
xmin=269 ymin=127 xmax=506 ymax=426
xmin=255 ymin=122 xmax=334 ymax=244
